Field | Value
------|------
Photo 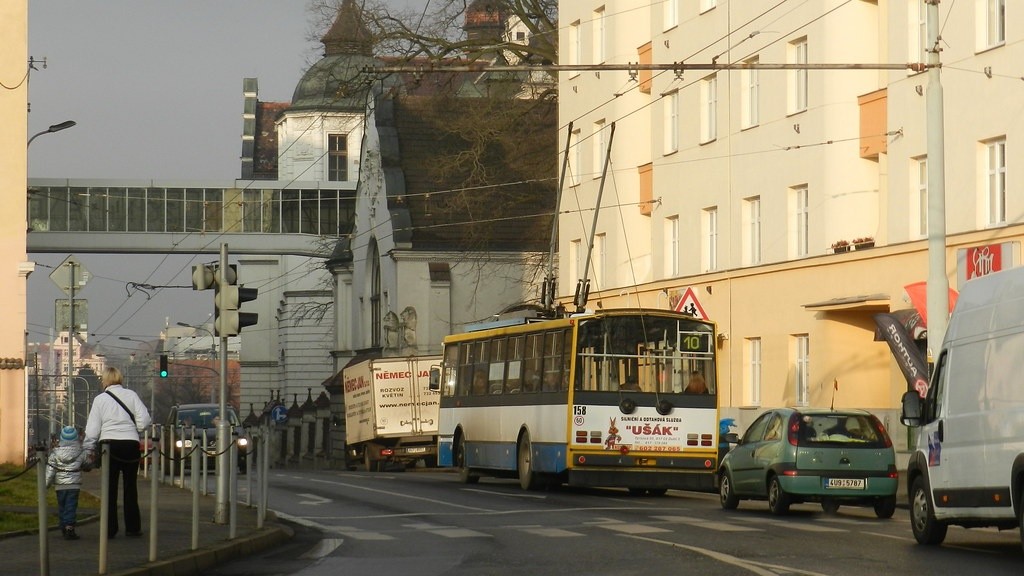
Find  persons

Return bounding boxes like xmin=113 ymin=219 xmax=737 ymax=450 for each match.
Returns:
xmin=46 ymin=425 xmax=95 ymax=539
xmin=82 ymin=367 xmax=152 ymax=540
xmin=684 ymin=371 xmax=709 ymax=394
xmin=618 ymin=376 xmax=641 ymax=391
xmin=183 ymin=415 xmax=194 ymax=427
xmin=212 ymin=414 xmax=220 ymax=427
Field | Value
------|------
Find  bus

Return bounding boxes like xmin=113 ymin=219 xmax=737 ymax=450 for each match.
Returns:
xmin=426 ymin=116 xmax=726 ymax=500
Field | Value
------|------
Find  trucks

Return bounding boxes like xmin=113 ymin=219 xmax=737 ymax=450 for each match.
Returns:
xmin=335 ymin=353 xmax=460 ymax=472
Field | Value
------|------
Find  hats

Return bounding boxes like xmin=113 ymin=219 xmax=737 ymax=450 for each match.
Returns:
xmin=60 ymin=425 xmax=78 ymax=441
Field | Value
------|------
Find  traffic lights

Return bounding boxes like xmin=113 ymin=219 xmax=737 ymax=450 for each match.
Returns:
xmin=158 ymin=353 xmax=171 ymax=379
xmin=223 ymin=283 xmax=261 ymax=333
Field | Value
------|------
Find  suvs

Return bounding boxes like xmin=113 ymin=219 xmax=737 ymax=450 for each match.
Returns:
xmin=162 ymin=403 xmax=254 ymax=475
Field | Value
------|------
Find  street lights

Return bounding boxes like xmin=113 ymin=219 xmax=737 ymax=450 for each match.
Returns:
xmin=178 ymin=321 xmax=217 ymax=403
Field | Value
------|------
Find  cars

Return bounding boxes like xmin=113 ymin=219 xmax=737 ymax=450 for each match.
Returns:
xmin=716 ymin=406 xmax=899 ymax=520
xmin=136 ymin=425 xmax=161 ymax=471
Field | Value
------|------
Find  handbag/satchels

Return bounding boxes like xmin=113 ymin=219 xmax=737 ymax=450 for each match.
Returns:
xmin=64 ymin=528 xmax=80 ymax=540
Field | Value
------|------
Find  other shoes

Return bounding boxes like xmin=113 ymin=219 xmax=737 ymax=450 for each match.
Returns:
xmin=126 ymin=529 xmax=144 ymax=537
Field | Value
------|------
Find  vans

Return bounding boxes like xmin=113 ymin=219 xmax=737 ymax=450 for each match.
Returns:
xmin=901 ymin=262 xmax=1024 ymax=547
